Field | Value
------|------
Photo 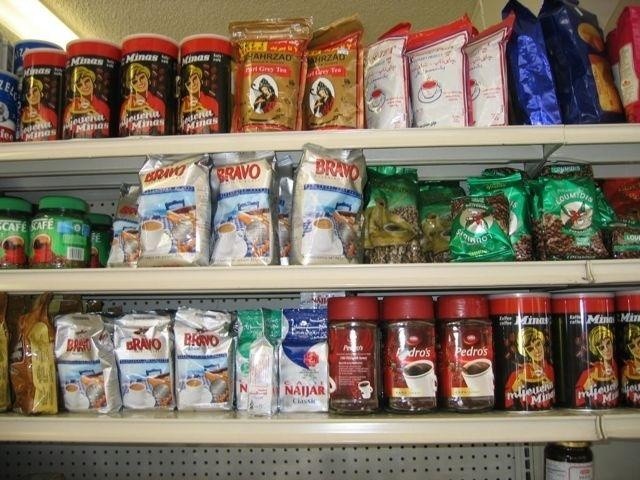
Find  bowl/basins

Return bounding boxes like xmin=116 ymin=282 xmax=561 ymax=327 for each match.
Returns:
xmin=383 ymin=222 xmax=410 ymax=237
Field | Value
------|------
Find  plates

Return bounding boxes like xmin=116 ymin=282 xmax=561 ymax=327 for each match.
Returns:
xmin=143 ymin=232 xmax=172 ymax=257
xmin=212 ymin=235 xmax=248 ymax=261
xmin=301 ymin=232 xmax=344 ymax=257
xmin=63 ymin=394 xmax=90 ymax=410
xmin=179 ymin=389 xmax=213 ymax=403
xmin=123 ymin=392 xmax=155 ymax=408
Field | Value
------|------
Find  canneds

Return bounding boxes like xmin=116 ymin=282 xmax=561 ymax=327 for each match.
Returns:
xmin=0 ymin=196 xmax=113 ymax=268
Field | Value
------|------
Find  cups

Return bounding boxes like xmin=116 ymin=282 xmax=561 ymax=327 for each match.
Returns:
xmin=2 ymin=234 xmax=53 ymax=265
xmin=358 ymin=381 xmax=373 ymax=399
xmin=64 ymin=384 xmax=86 ymax=407
xmin=141 ymin=219 xmax=172 ymax=251
xmin=91 ymin=245 xmax=101 ymax=268
xmin=462 ymin=358 xmax=494 ymax=397
xmin=128 ymin=382 xmax=152 ymax=405
xmin=312 ymin=217 xmax=338 ymax=252
xmin=403 ymin=360 xmax=438 ymax=397
xmin=184 ymin=378 xmax=209 ymax=402
xmin=367 ymin=79 xmax=480 ymax=114
xmin=217 ymin=223 xmax=241 ymax=254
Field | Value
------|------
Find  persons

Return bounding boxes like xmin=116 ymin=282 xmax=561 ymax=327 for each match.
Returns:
xmin=253 ymin=78 xmax=277 ymax=114
xmin=177 ymin=64 xmax=219 ymax=135
xmin=62 ymin=65 xmax=111 ymax=139
xmin=507 ymin=326 xmax=556 ymax=408
xmin=620 ymin=322 xmax=640 ymax=408
xmin=576 ymin=324 xmax=620 ymax=408
xmin=119 ymin=62 xmax=167 ymax=135
xmin=21 ymin=75 xmax=58 ymax=139
xmin=314 ymin=81 xmax=336 ymax=118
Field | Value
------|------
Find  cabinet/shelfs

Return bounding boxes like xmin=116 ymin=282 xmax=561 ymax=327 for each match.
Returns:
xmin=0 ymin=119 xmax=640 ymax=447
